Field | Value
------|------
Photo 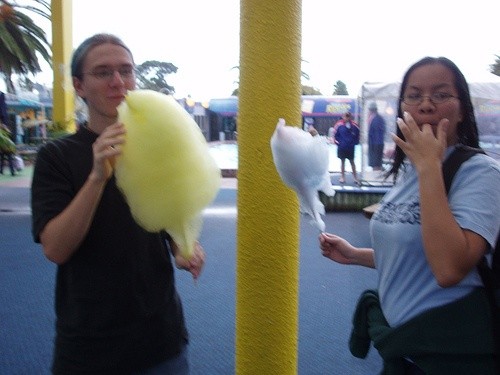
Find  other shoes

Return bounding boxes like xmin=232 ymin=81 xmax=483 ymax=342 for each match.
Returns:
xmin=354 ymin=176 xmax=359 ymax=182
xmin=340 ymin=177 xmax=344 ymax=183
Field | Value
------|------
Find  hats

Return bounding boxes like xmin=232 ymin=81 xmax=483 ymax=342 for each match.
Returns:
xmin=368 ymin=102 xmax=377 ymax=109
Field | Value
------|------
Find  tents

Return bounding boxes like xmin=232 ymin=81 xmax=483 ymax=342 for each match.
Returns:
xmin=358 ymin=79 xmax=500 ymax=188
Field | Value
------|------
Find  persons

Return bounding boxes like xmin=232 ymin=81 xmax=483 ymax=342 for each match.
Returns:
xmin=318 ymin=56 xmax=500 ymax=375
xmin=332 ymin=112 xmax=365 ymax=186
xmin=367 ymin=102 xmax=389 ymax=178
xmin=31 ymin=33 xmax=206 ymax=375
xmin=0 ymin=114 xmax=40 ymax=176
xmin=308 ymin=124 xmax=318 ymax=137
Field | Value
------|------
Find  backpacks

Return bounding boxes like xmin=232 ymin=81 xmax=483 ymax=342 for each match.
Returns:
xmin=489 ymin=231 xmax=500 ymax=327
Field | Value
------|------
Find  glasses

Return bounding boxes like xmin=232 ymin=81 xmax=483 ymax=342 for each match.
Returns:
xmin=400 ymin=92 xmax=461 ymax=106
xmin=76 ymin=67 xmax=136 ymax=81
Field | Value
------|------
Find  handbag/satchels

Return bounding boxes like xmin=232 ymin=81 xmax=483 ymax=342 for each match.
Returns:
xmin=14 ymin=156 xmax=24 ymax=171
